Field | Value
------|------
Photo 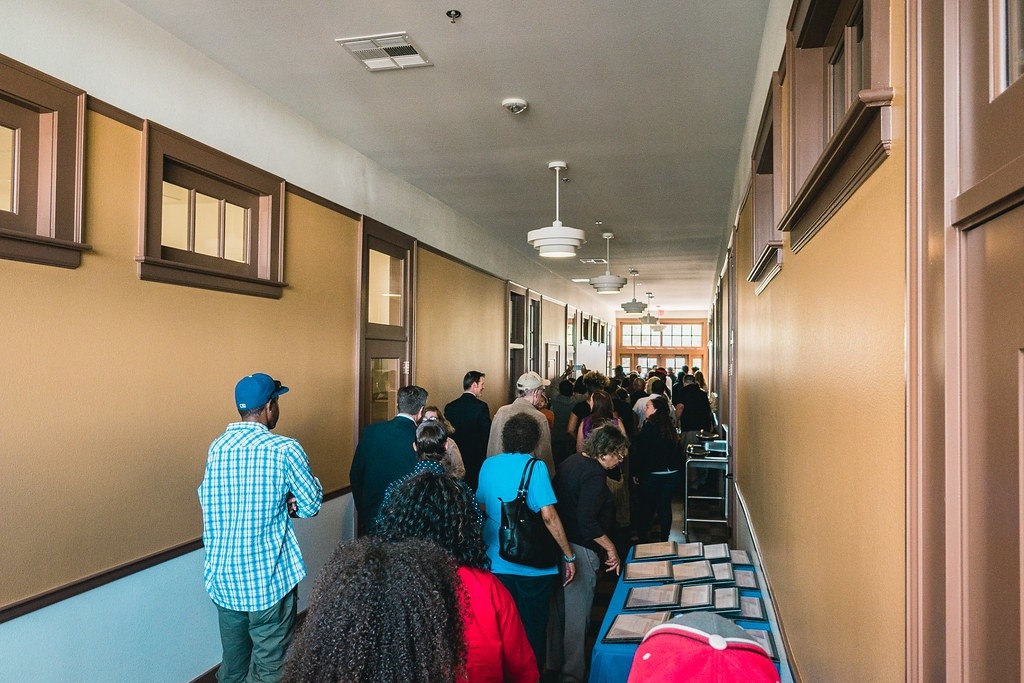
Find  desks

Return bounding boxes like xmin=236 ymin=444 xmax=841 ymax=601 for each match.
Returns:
xmin=591 ymin=546 xmax=782 ymax=683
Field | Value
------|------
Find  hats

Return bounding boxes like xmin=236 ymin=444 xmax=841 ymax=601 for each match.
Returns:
xmin=235 ymin=373 xmax=288 ymax=410
xmin=516 ymin=371 xmax=551 ymax=390
xmin=627 ymin=612 xmax=781 ymax=683
xmin=657 ymin=369 xmax=668 ymax=375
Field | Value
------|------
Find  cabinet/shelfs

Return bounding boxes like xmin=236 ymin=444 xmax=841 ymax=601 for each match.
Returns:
xmin=683 ymin=440 xmax=730 ymax=535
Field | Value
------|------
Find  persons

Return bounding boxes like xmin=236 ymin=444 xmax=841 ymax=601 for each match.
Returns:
xmin=376 ymin=472 xmax=539 ymax=683
xmin=421 ymin=405 xmax=466 ymax=479
xmin=486 ymin=372 xmax=555 ymax=478
xmin=535 ymin=365 xmax=716 ymax=555
xmin=444 ymin=371 xmax=491 ymax=489
xmin=628 ymin=612 xmax=780 ymax=683
xmin=378 ymin=419 xmax=485 ymax=528
xmin=350 ymin=385 xmax=429 ymax=538
xmin=475 ymin=413 xmax=576 ymax=683
xmin=279 ymin=538 xmax=466 ymax=683
xmin=555 ymin=426 xmax=627 ymax=683
xmin=197 ymin=373 xmax=325 ymax=683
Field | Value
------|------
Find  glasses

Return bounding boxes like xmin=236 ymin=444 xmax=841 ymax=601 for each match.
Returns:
xmin=617 ymin=451 xmax=625 ymax=462
xmin=422 ymin=417 xmax=439 ymax=421
xmin=268 ymin=380 xmax=281 ymax=401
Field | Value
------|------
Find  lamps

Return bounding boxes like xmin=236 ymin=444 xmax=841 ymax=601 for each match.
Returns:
xmin=590 ymin=232 xmax=627 ymax=294
xmin=639 ymin=292 xmax=658 ymax=325
xmin=622 ymin=268 xmax=647 ymax=313
xmin=650 ymin=305 xmax=667 ymax=332
xmin=528 ymin=160 xmax=587 ymax=258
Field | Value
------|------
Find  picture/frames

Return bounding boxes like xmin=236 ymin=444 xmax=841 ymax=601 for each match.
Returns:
xmin=601 ymin=542 xmax=780 ymax=662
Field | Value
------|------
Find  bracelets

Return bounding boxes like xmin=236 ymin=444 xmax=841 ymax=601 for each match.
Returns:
xmin=564 ymin=553 xmax=576 ymax=562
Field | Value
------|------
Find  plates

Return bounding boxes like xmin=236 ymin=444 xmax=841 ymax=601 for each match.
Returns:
xmin=695 ymin=432 xmax=719 ymax=440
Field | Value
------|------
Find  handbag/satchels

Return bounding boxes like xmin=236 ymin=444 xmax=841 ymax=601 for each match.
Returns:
xmin=498 ymin=458 xmax=562 ymax=569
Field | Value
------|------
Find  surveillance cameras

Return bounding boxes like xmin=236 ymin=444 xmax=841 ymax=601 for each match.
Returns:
xmin=501 ymin=98 xmax=528 ymax=115
